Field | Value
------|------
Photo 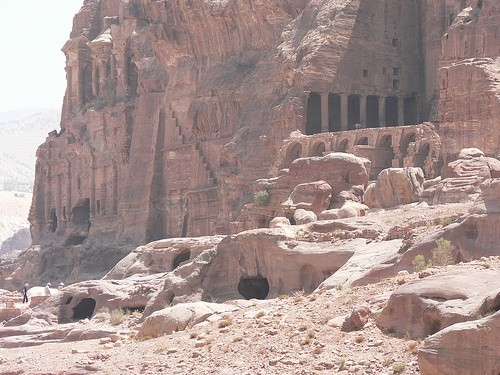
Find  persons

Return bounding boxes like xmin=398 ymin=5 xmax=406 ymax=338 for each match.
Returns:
xmin=45 ymin=282 xmax=53 ymax=297
xmin=58 ymin=281 xmax=65 ymax=291
xmin=23 ymin=282 xmax=29 ymax=303
